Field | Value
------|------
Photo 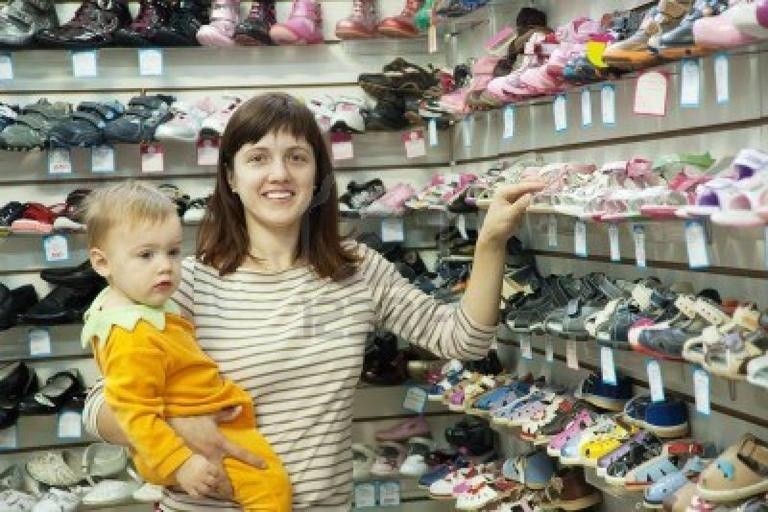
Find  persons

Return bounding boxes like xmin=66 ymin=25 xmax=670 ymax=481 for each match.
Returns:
xmin=81 ymin=89 xmax=546 ymax=511
xmin=81 ymin=177 xmax=294 ymax=511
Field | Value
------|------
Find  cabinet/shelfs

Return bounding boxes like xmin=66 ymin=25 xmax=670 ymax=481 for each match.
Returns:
xmin=0 ymin=1 xmax=768 ymax=512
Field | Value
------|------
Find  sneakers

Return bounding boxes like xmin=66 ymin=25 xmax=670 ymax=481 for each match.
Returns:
xmin=0 ymin=0 xmax=768 ymax=511
